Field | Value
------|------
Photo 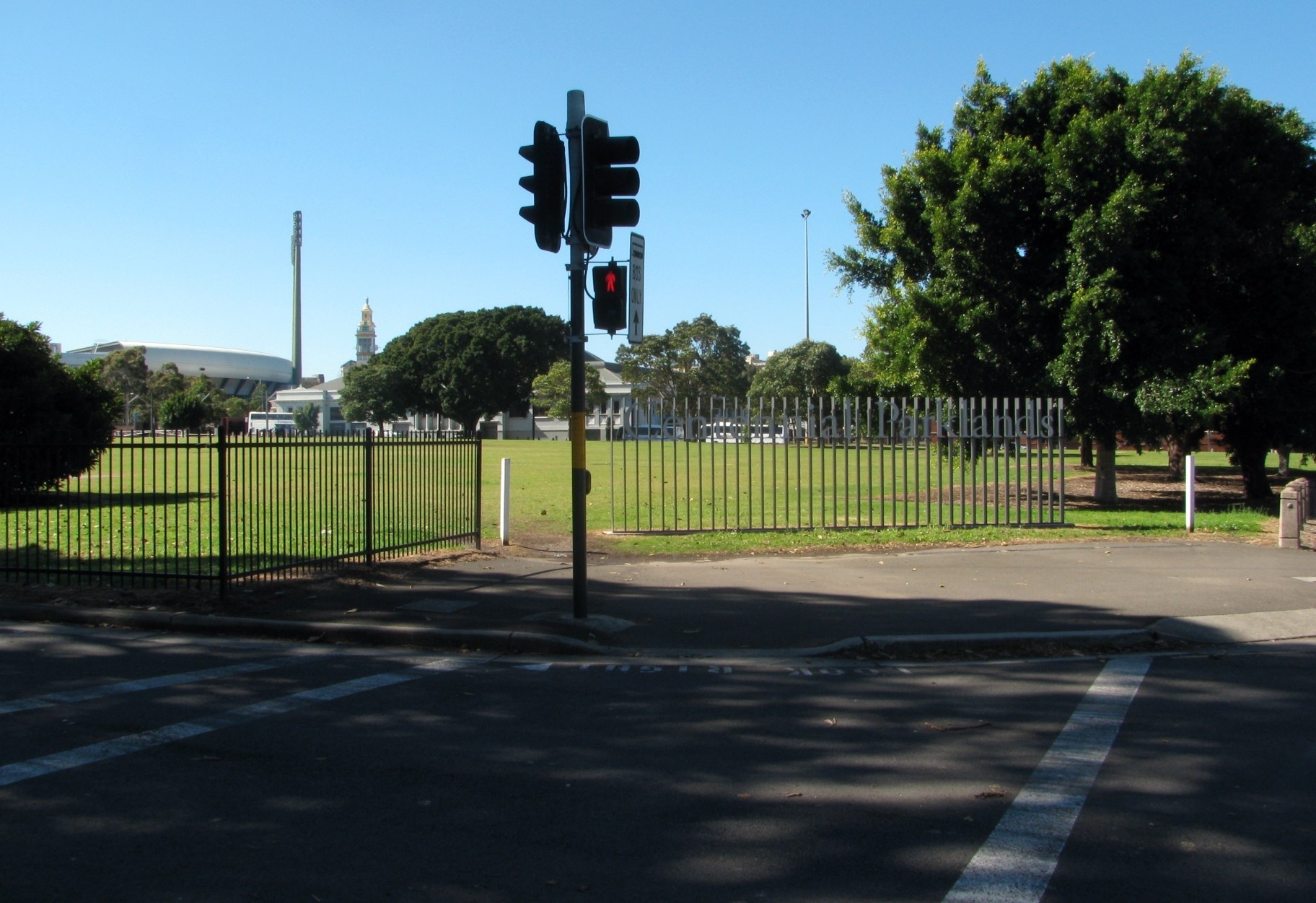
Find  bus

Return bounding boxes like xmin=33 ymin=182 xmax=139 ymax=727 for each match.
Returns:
xmin=695 ymin=422 xmax=787 ymax=444
xmin=248 ymin=411 xmax=309 ymax=436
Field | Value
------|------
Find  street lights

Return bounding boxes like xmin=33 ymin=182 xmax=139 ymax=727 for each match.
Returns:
xmin=801 ymin=208 xmax=813 ymax=342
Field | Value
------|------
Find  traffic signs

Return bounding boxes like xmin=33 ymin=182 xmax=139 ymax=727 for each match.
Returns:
xmin=629 ymin=232 xmax=647 ymax=345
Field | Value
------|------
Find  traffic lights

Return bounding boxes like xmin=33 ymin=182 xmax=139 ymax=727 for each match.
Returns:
xmin=579 ymin=114 xmax=640 ymax=248
xmin=591 ymin=266 xmax=627 ymax=330
xmin=518 ymin=121 xmax=565 ymax=254
